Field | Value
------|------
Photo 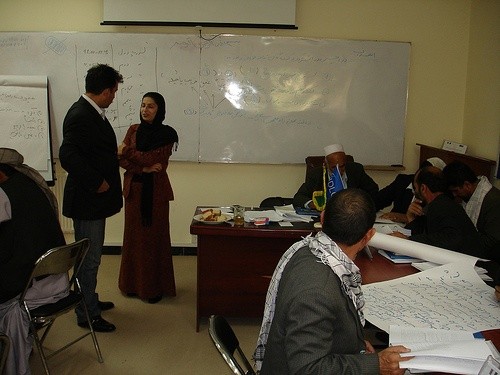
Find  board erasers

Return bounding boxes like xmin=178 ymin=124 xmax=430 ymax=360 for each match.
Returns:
xmin=391 ymin=165 xmax=403 ymax=167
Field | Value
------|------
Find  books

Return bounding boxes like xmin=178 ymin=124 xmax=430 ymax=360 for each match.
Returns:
xmin=388 ymin=325 xmax=500 ymax=375
xmin=378 ymin=247 xmax=494 ymax=283
xmin=372 ymin=211 xmax=413 ymax=237
xmin=242 ymin=205 xmax=322 ymax=223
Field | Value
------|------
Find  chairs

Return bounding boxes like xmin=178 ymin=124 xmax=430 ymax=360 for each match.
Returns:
xmin=208 ymin=314 xmax=256 ymax=375
xmin=0 ymin=238 xmax=104 ymax=374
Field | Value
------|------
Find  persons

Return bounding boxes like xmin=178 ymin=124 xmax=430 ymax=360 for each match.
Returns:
xmin=294 ymin=144 xmax=380 ymax=209
xmin=494 ymin=286 xmax=500 ymax=301
xmin=259 ymin=189 xmax=414 ymax=375
xmin=117 ymin=92 xmax=179 ymax=304
xmin=60 ymin=63 xmax=124 ymax=332
xmin=378 ymin=156 xmax=446 ymax=221
xmin=0 ymin=147 xmax=67 ymax=375
xmin=386 ymin=166 xmax=491 ymax=260
xmin=442 ymin=161 xmax=500 ymax=257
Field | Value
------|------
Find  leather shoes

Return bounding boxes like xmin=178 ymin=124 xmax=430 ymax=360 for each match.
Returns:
xmin=99 ymin=301 xmax=114 ymax=311
xmin=77 ymin=317 xmax=116 ymax=332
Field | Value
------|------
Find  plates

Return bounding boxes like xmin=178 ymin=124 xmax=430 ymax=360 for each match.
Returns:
xmin=193 ymin=214 xmax=232 ymax=225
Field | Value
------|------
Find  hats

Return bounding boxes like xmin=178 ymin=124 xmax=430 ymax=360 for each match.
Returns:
xmin=427 ymin=158 xmax=446 ymax=170
xmin=324 ymin=144 xmax=344 ymax=157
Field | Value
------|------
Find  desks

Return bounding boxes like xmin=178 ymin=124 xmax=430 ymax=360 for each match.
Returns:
xmin=353 ymin=245 xmax=500 ymax=374
xmin=190 ymin=205 xmax=321 ymax=333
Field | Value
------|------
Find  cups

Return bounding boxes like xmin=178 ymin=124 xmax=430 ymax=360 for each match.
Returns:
xmin=234 ymin=208 xmax=245 ymax=226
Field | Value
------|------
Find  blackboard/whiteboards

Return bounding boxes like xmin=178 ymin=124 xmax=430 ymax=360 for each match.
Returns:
xmin=0 ymin=29 xmax=412 ymax=171
xmin=0 ymin=75 xmax=57 ymax=187
